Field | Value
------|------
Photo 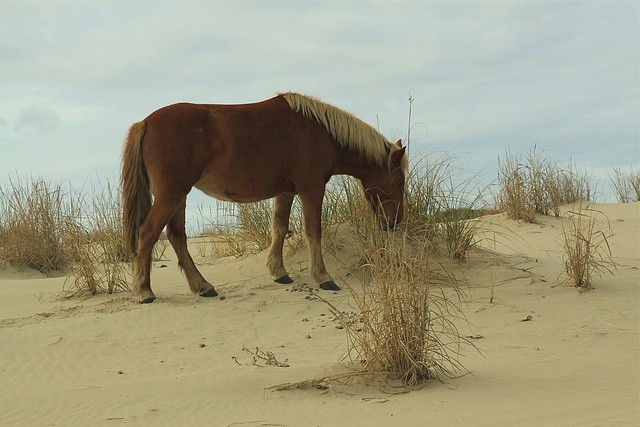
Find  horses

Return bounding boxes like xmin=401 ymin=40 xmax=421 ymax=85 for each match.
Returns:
xmin=120 ymin=91 xmax=409 ymax=306
